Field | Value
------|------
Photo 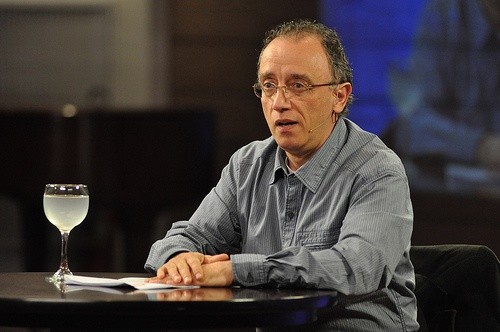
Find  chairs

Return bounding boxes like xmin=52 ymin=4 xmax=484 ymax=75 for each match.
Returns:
xmin=409 ymin=244 xmax=500 ymax=332
xmin=0 ymin=106 xmax=221 ymax=272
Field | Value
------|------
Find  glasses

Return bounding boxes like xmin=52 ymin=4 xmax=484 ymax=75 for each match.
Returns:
xmin=252 ymin=78 xmax=337 ymax=98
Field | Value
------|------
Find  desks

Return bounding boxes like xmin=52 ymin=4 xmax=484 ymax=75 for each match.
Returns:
xmin=0 ymin=272 xmax=338 ymax=332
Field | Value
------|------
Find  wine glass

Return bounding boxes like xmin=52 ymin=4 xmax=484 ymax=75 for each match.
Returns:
xmin=43 ymin=183 xmax=89 ymax=285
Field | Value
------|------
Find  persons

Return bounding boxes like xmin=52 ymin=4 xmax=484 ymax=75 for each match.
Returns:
xmin=390 ymin=0 xmax=499 ymax=166
xmin=144 ymin=20 xmax=420 ymax=332
